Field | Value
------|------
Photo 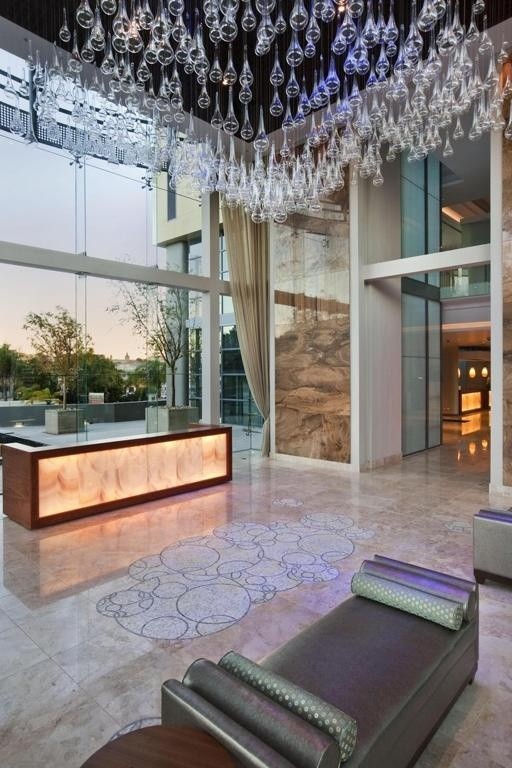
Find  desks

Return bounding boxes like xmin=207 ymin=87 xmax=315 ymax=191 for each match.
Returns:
xmin=75 ymin=719 xmax=241 ymax=766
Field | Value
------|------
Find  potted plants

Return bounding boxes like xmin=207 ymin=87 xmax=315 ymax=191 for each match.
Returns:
xmin=17 ymin=303 xmax=86 ymax=433
xmin=105 ymin=261 xmax=201 ymax=434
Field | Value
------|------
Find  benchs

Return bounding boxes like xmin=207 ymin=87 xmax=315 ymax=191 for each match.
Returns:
xmin=161 ymin=550 xmax=481 ymax=766
xmin=472 ymin=501 xmax=512 ymax=590
